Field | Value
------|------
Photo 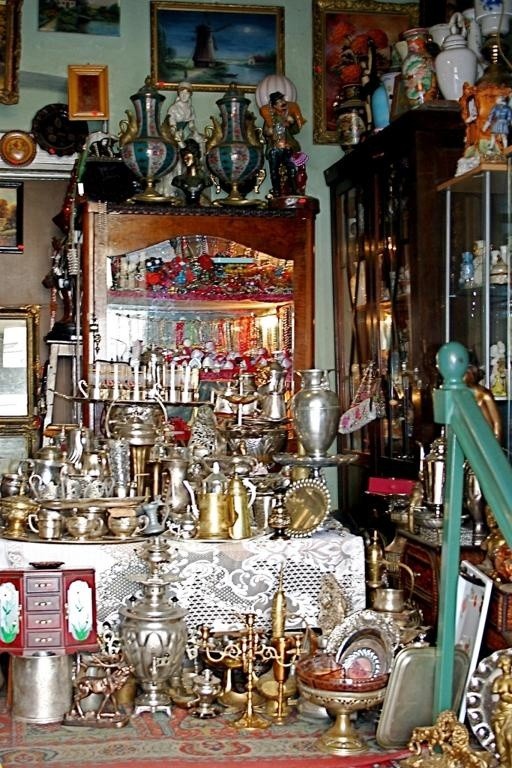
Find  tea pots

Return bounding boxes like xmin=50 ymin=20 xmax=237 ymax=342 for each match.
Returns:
xmin=158 ymin=440 xmax=287 ymax=548
xmin=415 ymin=424 xmax=448 ymax=519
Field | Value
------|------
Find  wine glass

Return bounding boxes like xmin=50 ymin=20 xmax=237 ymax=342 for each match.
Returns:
xmin=297 ymin=658 xmax=392 ymax=758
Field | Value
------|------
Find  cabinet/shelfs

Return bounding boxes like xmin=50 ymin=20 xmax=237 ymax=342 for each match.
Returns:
xmin=322 ymin=101 xmax=482 ymax=660
xmin=77 ymin=198 xmax=318 ymax=431
xmin=0 ymin=533 xmax=375 ymax=710
xmin=436 ymin=162 xmax=512 ymax=403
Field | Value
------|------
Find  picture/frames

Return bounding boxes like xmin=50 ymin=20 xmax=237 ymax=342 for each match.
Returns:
xmin=0 ymin=308 xmax=43 ymax=429
xmin=312 ymin=0 xmax=418 ymax=146
xmin=68 ymin=64 xmax=109 ymax=121
xmin=0 ymin=178 xmax=23 ymax=254
xmin=150 ymin=1 xmax=285 ymax=93
xmin=0 ymin=0 xmax=25 ymax=104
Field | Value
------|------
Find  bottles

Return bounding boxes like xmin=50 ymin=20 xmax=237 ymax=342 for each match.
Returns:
xmin=213 ymin=362 xmax=288 ymax=421
xmin=464 ymin=467 xmax=489 ymax=548
xmin=459 ymin=237 xmax=511 ymax=290
xmin=291 ymin=367 xmax=346 ymax=461
xmin=118 ymin=77 xmax=177 ymax=203
xmin=365 ymin=529 xmax=384 ymax=586
xmin=201 ymin=80 xmax=265 ymax=209
xmin=330 ymin=0 xmax=512 ymax=151
xmin=81 ymin=358 xmax=200 ymax=406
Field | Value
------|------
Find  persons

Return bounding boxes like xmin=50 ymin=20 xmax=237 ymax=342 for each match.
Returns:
xmin=260 ymin=92 xmax=306 ymax=197
xmin=154 ymin=82 xmax=201 ymax=198
xmin=491 ymin=655 xmax=512 ymax=768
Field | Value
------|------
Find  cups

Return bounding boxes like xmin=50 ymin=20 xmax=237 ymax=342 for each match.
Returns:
xmin=0 ymin=428 xmax=133 ymax=500
xmin=0 ymin=497 xmax=164 ymax=541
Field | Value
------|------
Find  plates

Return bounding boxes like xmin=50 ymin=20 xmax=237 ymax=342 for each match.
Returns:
xmin=30 ymin=98 xmax=90 ymax=156
xmin=2 ymin=131 xmax=39 ymax=164
